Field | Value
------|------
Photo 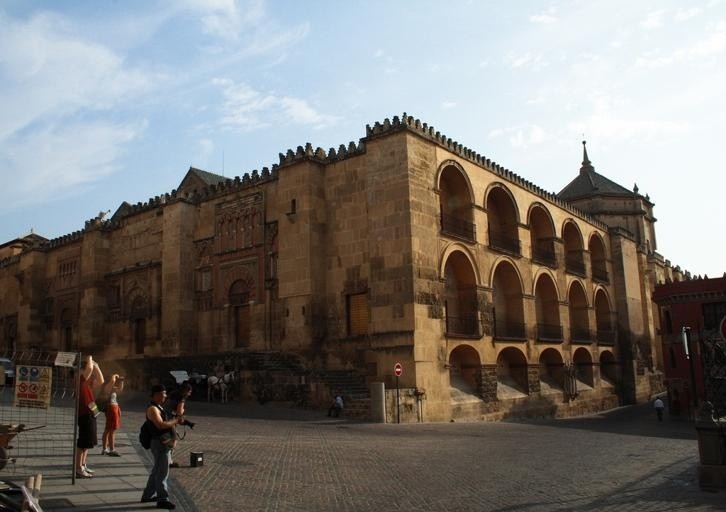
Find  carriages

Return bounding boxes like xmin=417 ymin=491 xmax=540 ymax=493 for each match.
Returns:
xmin=169 ymin=367 xmax=239 ymax=405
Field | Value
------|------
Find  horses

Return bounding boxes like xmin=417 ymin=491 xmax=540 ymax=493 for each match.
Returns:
xmin=206 ymin=369 xmax=236 ymax=403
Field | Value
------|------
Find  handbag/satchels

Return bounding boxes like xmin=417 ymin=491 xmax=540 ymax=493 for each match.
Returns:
xmin=139 ymin=419 xmax=152 ymax=449
xmin=88 ymin=401 xmax=101 ymax=418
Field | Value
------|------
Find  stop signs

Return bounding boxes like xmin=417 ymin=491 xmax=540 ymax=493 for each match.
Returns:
xmin=393 ymin=362 xmax=405 ymax=379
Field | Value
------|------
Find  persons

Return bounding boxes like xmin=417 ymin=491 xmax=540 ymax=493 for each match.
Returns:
xmin=100 ymin=371 xmax=124 ymax=457
xmin=326 ymin=393 xmax=345 ymax=418
xmin=73 ymin=356 xmax=105 ymax=479
xmin=164 ymin=383 xmax=194 ymax=469
xmin=653 ymin=396 xmax=666 ymax=422
xmin=140 ymin=384 xmax=187 ymax=512
xmin=189 ymin=367 xmax=204 ymax=385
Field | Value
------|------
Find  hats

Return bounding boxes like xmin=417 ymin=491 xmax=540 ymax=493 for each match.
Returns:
xmin=152 ymin=384 xmax=166 ymax=393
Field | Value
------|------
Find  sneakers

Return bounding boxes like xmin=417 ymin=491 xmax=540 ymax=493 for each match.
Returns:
xmin=140 ymin=495 xmax=176 ymax=510
xmin=75 ymin=464 xmax=95 ymax=478
xmin=102 ymin=448 xmax=121 ymax=458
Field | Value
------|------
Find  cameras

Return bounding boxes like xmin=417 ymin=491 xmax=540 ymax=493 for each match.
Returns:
xmin=180 ymin=419 xmax=196 ymax=429
